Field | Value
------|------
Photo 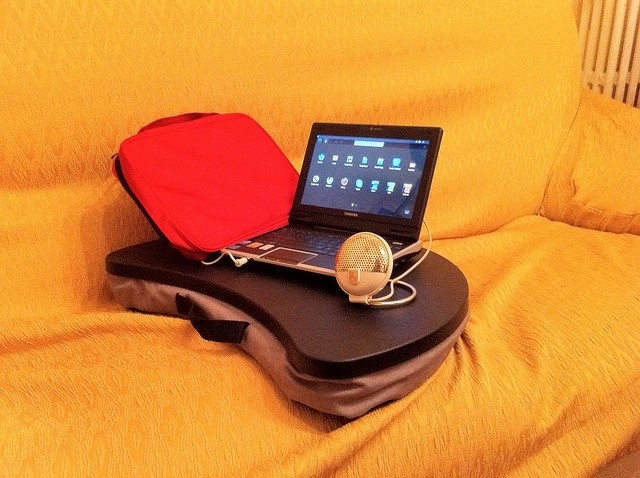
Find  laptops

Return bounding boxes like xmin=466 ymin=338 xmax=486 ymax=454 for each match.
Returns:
xmin=219 ymin=121 xmax=444 ymax=278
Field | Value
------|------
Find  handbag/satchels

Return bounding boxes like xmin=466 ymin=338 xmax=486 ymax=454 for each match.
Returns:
xmin=112 ymin=112 xmax=302 ymax=261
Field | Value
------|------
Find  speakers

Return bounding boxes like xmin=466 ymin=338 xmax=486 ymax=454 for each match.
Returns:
xmin=335 ymin=231 xmax=394 ymax=307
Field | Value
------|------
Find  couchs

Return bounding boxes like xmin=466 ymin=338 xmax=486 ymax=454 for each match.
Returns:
xmin=0 ymin=0 xmax=639 ymax=478
